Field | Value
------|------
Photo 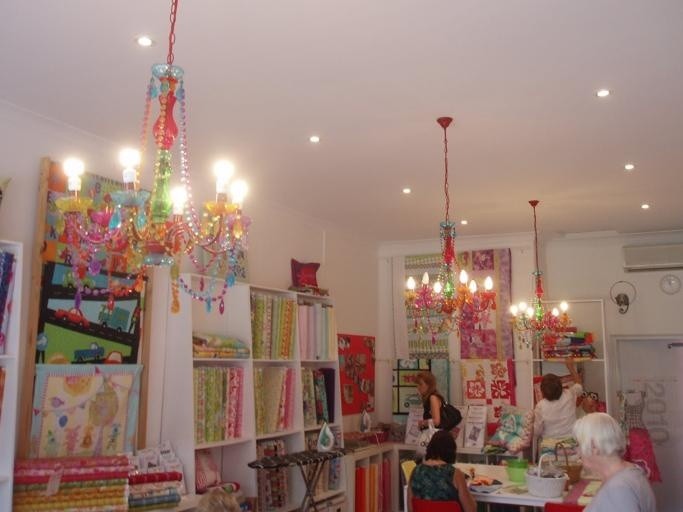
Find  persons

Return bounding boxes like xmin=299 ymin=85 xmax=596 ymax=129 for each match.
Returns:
xmin=572 ymin=411 xmax=657 ymax=512
xmin=406 ymin=429 xmax=478 ymax=511
xmin=532 ymin=356 xmax=586 ymax=465
xmin=414 ymin=369 xmax=447 ymax=432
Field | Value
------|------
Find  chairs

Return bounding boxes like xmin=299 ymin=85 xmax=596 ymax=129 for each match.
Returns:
xmin=401 ymin=460 xmax=416 ymax=512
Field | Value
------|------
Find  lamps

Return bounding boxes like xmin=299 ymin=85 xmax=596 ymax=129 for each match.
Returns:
xmin=52 ymin=1 xmax=254 ymax=325
xmin=402 ymin=115 xmax=497 ymax=344
xmin=508 ymin=201 xmax=570 ymax=350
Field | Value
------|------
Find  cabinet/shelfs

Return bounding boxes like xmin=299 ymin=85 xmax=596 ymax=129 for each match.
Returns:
xmin=158 ymin=270 xmax=346 ymax=512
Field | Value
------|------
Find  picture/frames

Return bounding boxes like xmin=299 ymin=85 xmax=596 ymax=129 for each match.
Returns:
xmin=28 ymin=260 xmax=149 ymax=457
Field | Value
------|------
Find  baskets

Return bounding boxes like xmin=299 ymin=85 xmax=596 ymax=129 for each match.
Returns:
xmin=551 ymin=443 xmax=583 ymax=482
xmin=525 ymin=453 xmax=568 ymax=497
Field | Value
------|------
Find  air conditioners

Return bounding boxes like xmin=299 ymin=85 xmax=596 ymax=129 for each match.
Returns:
xmin=622 ymin=243 xmax=682 ymax=270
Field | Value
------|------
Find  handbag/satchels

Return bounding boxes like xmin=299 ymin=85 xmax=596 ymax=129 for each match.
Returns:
xmin=440 ymin=403 xmax=462 ymax=431
xmin=416 ymin=419 xmax=440 ymax=456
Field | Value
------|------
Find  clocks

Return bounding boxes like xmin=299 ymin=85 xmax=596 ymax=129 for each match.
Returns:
xmin=660 ymin=273 xmax=681 ymax=295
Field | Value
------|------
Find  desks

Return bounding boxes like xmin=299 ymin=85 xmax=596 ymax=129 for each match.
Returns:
xmin=341 ymin=441 xmax=523 ymax=512
xmin=246 ymin=449 xmax=356 ymax=512
xmin=454 ymin=462 xmax=575 ymax=511
